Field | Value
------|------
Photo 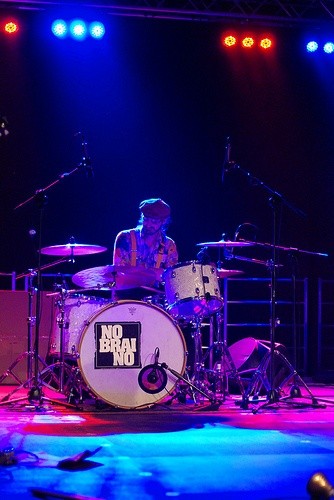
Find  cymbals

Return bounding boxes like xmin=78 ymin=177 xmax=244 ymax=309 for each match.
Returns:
xmin=198 ymin=241 xmax=253 ymax=247
xmin=37 ymin=243 xmax=106 ymax=256
xmin=215 ymin=267 xmax=242 ymax=276
xmin=72 ymin=265 xmax=156 ymax=290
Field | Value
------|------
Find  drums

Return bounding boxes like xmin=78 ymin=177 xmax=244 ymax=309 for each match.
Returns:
xmin=159 ymin=259 xmax=223 ymax=319
xmin=72 ymin=298 xmax=189 ymax=410
xmin=49 ymin=293 xmax=114 ymax=358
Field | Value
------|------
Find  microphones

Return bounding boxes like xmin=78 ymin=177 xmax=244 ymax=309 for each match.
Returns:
xmin=224 ymin=137 xmax=231 ymax=172
xmin=231 ymin=225 xmax=242 ymax=255
xmin=82 ymin=141 xmax=94 ymax=177
xmin=147 ymin=349 xmax=159 ymax=384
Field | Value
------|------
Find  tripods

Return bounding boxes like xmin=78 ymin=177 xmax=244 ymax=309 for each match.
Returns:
xmin=189 ymin=162 xmax=327 ymax=410
xmin=0 ymin=163 xmax=96 ymax=411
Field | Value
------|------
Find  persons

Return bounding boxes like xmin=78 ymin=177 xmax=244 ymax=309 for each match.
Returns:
xmin=113 ymin=198 xmax=178 ymax=311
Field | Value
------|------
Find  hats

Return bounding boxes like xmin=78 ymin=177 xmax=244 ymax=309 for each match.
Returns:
xmin=139 ymin=198 xmax=170 ymax=219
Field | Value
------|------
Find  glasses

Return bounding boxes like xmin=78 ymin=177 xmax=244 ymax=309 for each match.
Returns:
xmin=143 ymin=217 xmax=162 ymax=223
